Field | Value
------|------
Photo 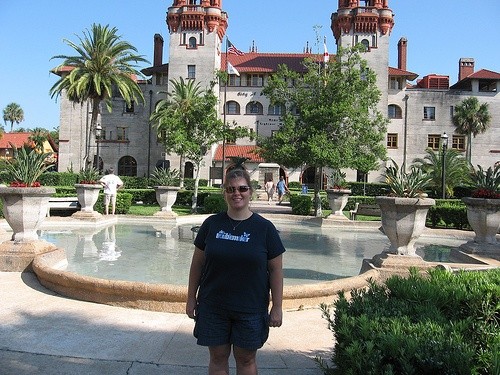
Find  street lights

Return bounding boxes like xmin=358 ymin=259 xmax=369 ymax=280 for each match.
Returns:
xmin=95 ymin=123 xmax=103 ymax=179
xmin=440 ymin=132 xmax=449 ymax=198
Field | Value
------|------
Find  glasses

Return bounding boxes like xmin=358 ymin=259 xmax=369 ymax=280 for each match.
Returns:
xmin=225 ymin=185 xmax=251 ymax=193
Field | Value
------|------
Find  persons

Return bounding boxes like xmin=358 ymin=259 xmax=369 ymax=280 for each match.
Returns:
xmin=265 ymin=179 xmax=275 ymax=205
xmin=100 ymin=167 xmax=123 ymax=216
xmin=186 ymin=169 xmax=287 ymax=375
xmin=276 ymin=176 xmax=288 ymax=205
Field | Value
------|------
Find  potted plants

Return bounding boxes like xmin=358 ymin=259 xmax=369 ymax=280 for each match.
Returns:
xmin=453 ymin=162 xmax=500 ymax=244
xmin=0 ymin=138 xmax=56 ymax=242
xmin=322 ymin=169 xmax=351 ymax=214
xmin=149 ymin=168 xmax=184 ymax=213
xmin=75 ymin=165 xmax=104 ymax=213
xmin=372 ymin=157 xmax=436 ymax=257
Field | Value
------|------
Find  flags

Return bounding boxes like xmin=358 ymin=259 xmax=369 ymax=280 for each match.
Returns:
xmin=323 ymin=44 xmax=329 ymax=70
xmin=228 ymin=40 xmax=244 ymax=56
xmin=228 ymin=62 xmax=240 ymax=76
